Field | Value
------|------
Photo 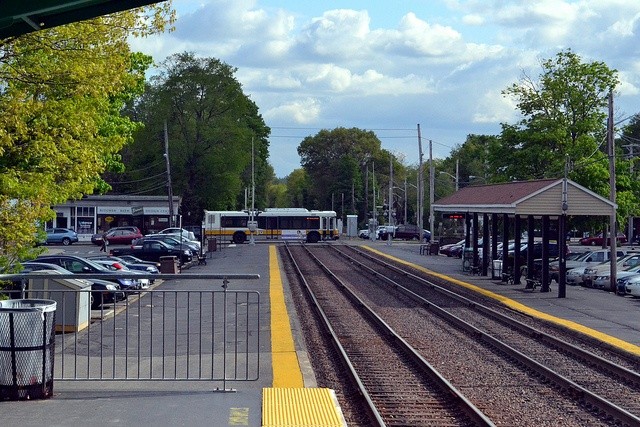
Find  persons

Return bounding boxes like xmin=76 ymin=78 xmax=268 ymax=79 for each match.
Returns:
xmin=100 ymin=230 xmax=108 ymax=252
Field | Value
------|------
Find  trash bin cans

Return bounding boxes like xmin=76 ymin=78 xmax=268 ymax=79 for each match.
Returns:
xmin=0 ymin=299 xmax=57 ymax=401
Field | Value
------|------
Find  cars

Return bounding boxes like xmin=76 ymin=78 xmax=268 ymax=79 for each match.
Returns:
xmin=379 ymin=224 xmax=421 ymax=240
xmin=582 ymin=252 xmax=640 ymax=282
xmin=145 ymin=226 xmax=196 ymax=241
xmin=421 ymin=228 xmax=431 ymax=238
xmin=84 ymin=255 xmax=160 ymax=282
xmin=581 ymin=231 xmax=627 ymax=246
xmin=1 ymin=261 xmax=123 ymax=309
xmin=617 ymin=272 xmax=640 ymax=297
xmin=37 ymin=227 xmax=78 ymax=246
xmin=21 ymin=254 xmax=140 ymax=294
xmin=591 ymin=263 xmax=640 ymax=289
xmin=439 ymin=234 xmax=586 ymax=282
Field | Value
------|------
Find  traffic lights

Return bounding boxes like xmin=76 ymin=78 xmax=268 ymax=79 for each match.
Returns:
xmin=259 ymin=211 xmax=262 ymax=213
xmin=244 ymin=210 xmax=249 ymax=213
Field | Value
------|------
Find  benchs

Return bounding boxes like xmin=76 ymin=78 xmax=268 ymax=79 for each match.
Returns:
xmin=420 ymin=240 xmax=438 ymax=256
xmin=501 ymin=267 xmax=524 ymax=285
xmin=526 ymin=270 xmax=553 ymax=292
xmin=469 ymin=258 xmax=490 ymax=276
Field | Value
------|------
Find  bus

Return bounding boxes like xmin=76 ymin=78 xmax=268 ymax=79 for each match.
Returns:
xmin=203 ymin=207 xmax=343 ymax=244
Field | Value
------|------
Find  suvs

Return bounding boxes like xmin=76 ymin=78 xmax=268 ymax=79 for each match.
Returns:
xmin=359 ymin=225 xmax=397 ymax=240
xmin=91 ymin=226 xmax=143 ymax=245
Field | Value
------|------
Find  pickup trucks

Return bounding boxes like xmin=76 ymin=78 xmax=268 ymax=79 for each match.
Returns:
xmin=110 ymin=238 xmax=193 ymax=265
